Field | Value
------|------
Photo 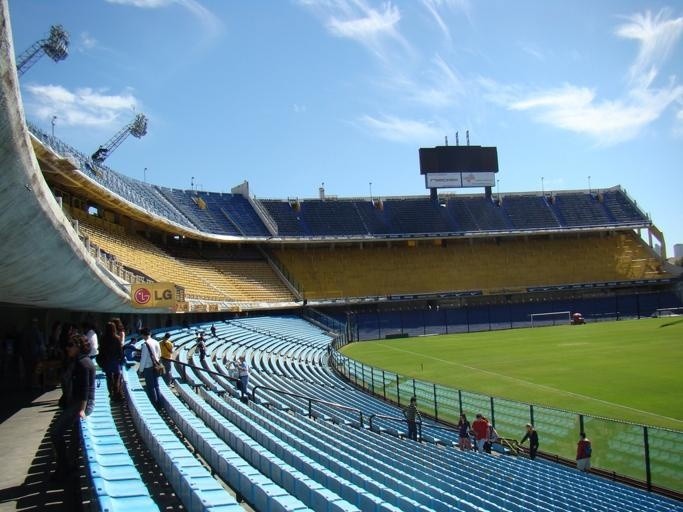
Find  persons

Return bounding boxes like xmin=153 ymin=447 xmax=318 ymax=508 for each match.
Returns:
xmin=572 ymin=432 xmax=591 ymax=472
xmin=454 ymin=412 xmax=471 ymax=452
xmin=235 ymin=356 xmax=249 ymax=392
xmin=401 ymin=396 xmax=422 ymax=442
xmin=520 ymin=422 xmax=538 ymax=460
xmin=210 ymin=322 xmax=216 ymax=336
xmin=0 ymin=313 xmax=174 ymax=404
xmin=481 ymin=417 xmax=496 ymax=454
xmin=194 ymin=330 xmax=206 ymax=360
xmin=470 ymin=413 xmax=489 ymax=453
xmin=49 ymin=333 xmax=96 ymax=480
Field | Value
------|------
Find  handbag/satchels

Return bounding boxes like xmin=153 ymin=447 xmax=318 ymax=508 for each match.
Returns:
xmin=152 ymin=360 xmax=166 ymax=377
